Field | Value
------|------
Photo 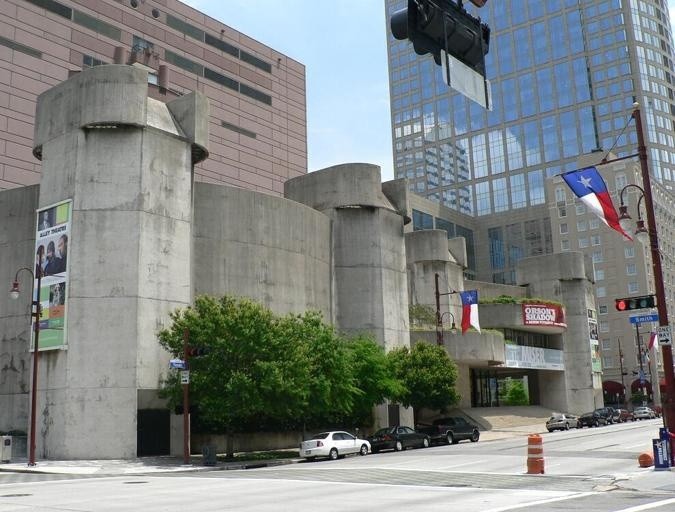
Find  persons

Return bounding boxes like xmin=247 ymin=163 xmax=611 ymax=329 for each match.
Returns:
xmin=42 ymin=241 xmax=64 ymax=275
xmin=55 ymin=234 xmax=67 ymax=273
xmin=38 ymin=211 xmax=52 ymax=231
xmin=35 ymin=245 xmax=44 ymax=279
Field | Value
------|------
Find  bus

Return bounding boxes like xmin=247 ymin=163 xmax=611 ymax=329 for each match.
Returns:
xmin=367 ymin=425 xmax=431 ymax=453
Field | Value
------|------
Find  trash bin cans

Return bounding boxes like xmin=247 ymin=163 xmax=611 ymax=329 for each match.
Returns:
xmin=0 ymin=435 xmax=12 ymax=464
xmin=202 ymin=443 xmax=217 ymax=466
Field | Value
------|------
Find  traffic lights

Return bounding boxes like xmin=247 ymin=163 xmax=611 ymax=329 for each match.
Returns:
xmin=391 ymin=1 xmax=491 ymax=69
xmin=616 ymin=295 xmax=656 ymax=312
xmin=186 ymin=345 xmax=213 ymax=357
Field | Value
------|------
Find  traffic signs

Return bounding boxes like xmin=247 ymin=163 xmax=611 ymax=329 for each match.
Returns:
xmin=180 ymin=369 xmax=190 ymax=385
xmin=658 ymin=326 xmax=672 ymax=346
xmin=630 ymin=315 xmax=659 ymax=323
xmin=168 ymin=359 xmax=185 ymax=369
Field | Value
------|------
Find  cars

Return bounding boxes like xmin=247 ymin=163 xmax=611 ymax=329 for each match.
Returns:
xmin=544 ymin=405 xmax=661 ymax=432
xmin=297 ymin=429 xmax=373 ymax=460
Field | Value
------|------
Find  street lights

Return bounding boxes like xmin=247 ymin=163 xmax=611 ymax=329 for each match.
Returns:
xmin=432 ymin=272 xmax=458 ymax=414
xmin=615 ymin=98 xmax=675 ymax=465
xmin=616 ymin=392 xmax=620 ymax=409
xmin=9 ymin=245 xmax=43 ymax=467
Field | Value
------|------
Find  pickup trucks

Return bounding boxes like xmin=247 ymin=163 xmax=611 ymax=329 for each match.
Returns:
xmin=418 ymin=417 xmax=480 ymax=446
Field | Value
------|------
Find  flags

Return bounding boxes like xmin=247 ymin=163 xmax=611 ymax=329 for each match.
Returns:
xmin=459 ymin=290 xmax=481 ymax=335
xmin=648 ymin=331 xmax=659 ymax=351
xmin=563 ymin=166 xmax=632 ymax=243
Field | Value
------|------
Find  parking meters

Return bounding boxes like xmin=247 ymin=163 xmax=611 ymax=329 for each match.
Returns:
xmin=354 ymin=428 xmax=359 ymax=456
